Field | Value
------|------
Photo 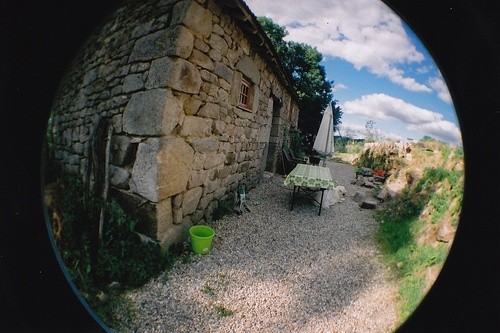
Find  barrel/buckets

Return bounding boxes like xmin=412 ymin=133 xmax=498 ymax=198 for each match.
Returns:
xmin=189 ymin=225 xmax=215 ymax=255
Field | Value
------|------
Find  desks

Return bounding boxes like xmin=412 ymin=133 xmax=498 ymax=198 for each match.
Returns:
xmin=284 ymin=164 xmax=333 ymax=217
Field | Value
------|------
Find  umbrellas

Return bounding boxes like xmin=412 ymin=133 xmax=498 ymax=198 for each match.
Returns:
xmin=312 ymin=104 xmax=335 ymax=167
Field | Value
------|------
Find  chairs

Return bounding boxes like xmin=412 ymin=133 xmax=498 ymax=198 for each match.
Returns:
xmin=282 ymin=149 xmax=299 ymax=175
xmin=289 ymin=149 xmax=306 ymax=166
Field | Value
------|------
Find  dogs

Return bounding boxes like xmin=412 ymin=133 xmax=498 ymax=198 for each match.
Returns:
xmin=311 ymin=186 xmax=346 ymax=207
